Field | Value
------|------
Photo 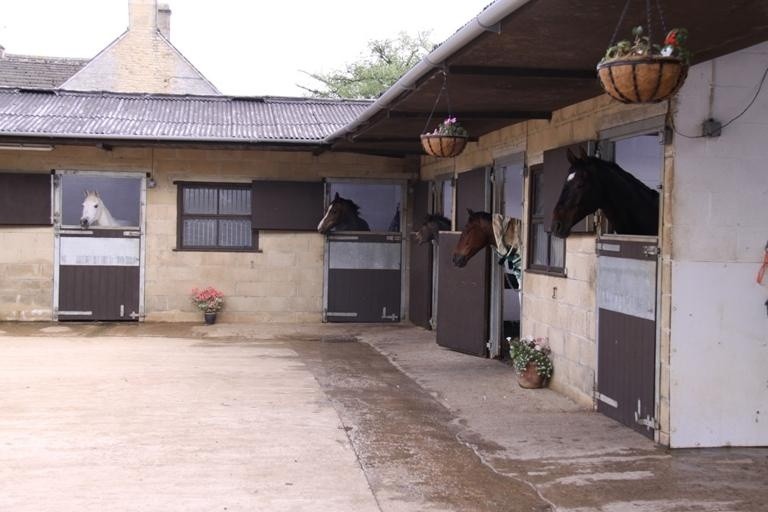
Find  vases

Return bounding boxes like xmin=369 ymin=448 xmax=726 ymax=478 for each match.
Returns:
xmin=514 ymin=360 xmax=548 ymax=389
xmin=420 ymin=133 xmax=466 ymax=157
xmin=594 ymin=58 xmax=690 ymax=104
xmin=205 ymin=312 xmax=216 ymax=324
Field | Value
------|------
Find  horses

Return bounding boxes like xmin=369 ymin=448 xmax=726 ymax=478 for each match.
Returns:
xmin=415 ymin=212 xmax=451 ymax=246
xmin=79 ymin=185 xmax=136 ymax=228
xmin=551 ymin=144 xmax=659 ymax=235
xmin=316 ymin=191 xmax=372 ymax=236
xmin=451 ymin=207 xmax=522 ymax=268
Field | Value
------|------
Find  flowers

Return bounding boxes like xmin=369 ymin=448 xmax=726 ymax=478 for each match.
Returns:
xmin=497 ymin=332 xmax=556 ymax=381
xmin=604 ymin=20 xmax=692 ymax=65
xmin=421 ymin=114 xmax=468 ymax=138
xmin=191 ymin=285 xmax=226 ymax=312
